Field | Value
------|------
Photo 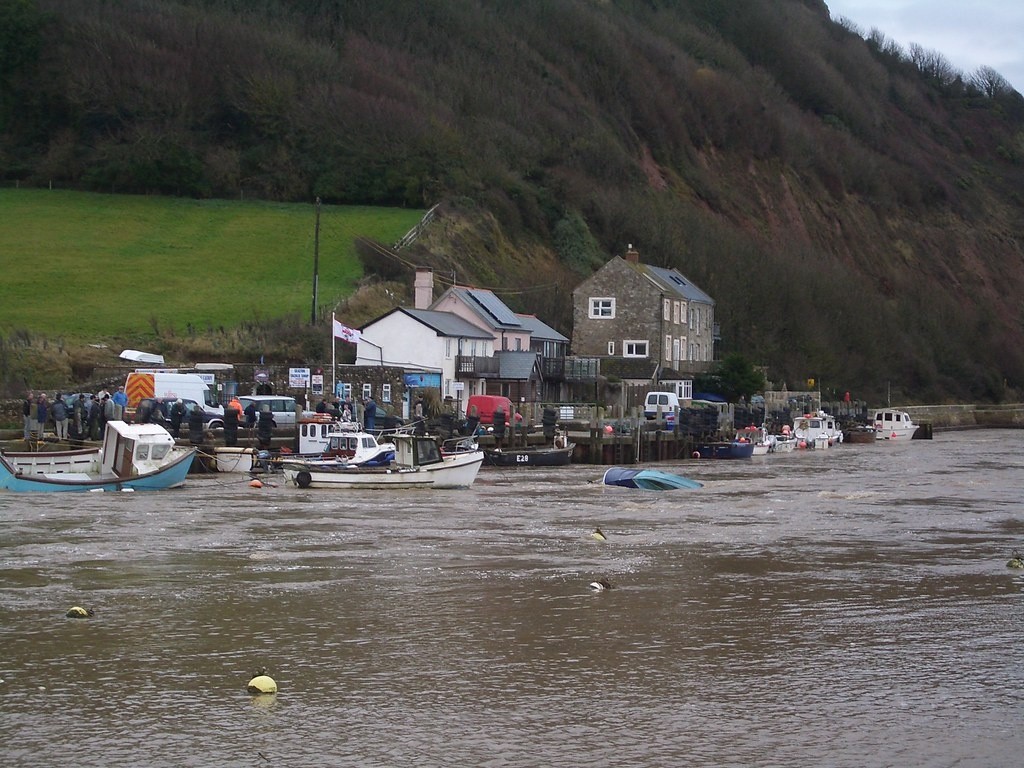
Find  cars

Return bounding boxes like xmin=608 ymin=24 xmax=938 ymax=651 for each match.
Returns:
xmin=349 ymin=400 xmax=407 ymax=428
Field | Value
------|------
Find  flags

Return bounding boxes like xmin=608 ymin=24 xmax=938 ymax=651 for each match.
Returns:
xmin=333 ymin=319 xmax=360 ymax=343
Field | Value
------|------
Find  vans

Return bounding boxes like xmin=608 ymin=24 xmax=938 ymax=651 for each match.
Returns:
xmin=125 ymin=373 xmax=223 ymax=428
xmin=466 ymin=395 xmax=524 ymax=424
xmin=645 ymin=393 xmax=680 ymax=431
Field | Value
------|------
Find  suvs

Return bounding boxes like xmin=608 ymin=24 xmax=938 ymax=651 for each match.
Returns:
xmin=237 ymin=394 xmax=317 ymax=429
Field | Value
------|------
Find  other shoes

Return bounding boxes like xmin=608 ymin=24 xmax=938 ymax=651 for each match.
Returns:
xmin=174 ymin=437 xmax=181 ymax=440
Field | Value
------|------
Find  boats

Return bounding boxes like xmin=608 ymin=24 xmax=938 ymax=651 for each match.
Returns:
xmin=440 ymin=437 xmax=578 ymax=468
xmin=0 ymin=419 xmax=201 ymax=494
xmin=691 ymin=408 xmax=846 ymax=459
xmin=601 ymin=467 xmax=703 ymax=492
xmin=856 ymin=408 xmax=920 ymax=441
xmin=250 ymin=422 xmax=485 ymax=491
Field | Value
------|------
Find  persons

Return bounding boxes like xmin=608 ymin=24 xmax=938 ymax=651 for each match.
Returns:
xmin=227 ymin=396 xmax=243 ymax=427
xmin=413 ymin=394 xmax=428 ymax=418
xmin=22 ymin=393 xmax=33 ymax=440
xmin=364 ymin=397 xmax=376 ymax=434
xmin=556 ymin=437 xmax=566 ymax=450
xmin=243 ymin=401 xmax=257 ymax=428
xmin=73 ymin=394 xmax=86 ymax=441
xmin=171 ymin=399 xmax=186 ymax=439
xmin=255 ymin=404 xmax=277 ymax=450
xmin=738 ymin=390 xmax=865 ymax=446
xmin=37 ymin=393 xmax=47 ymax=441
xmin=51 ymin=393 xmax=68 ymax=440
xmin=316 ymin=394 xmax=353 ymax=423
xmin=87 ymin=386 xmax=128 ymax=441
xmin=387 ymin=402 xmax=395 ymax=415
xmin=152 ymin=397 xmax=167 ymax=427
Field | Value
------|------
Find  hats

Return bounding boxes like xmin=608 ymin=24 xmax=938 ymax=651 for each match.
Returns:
xmin=119 ymin=386 xmax=124 ymax=389
